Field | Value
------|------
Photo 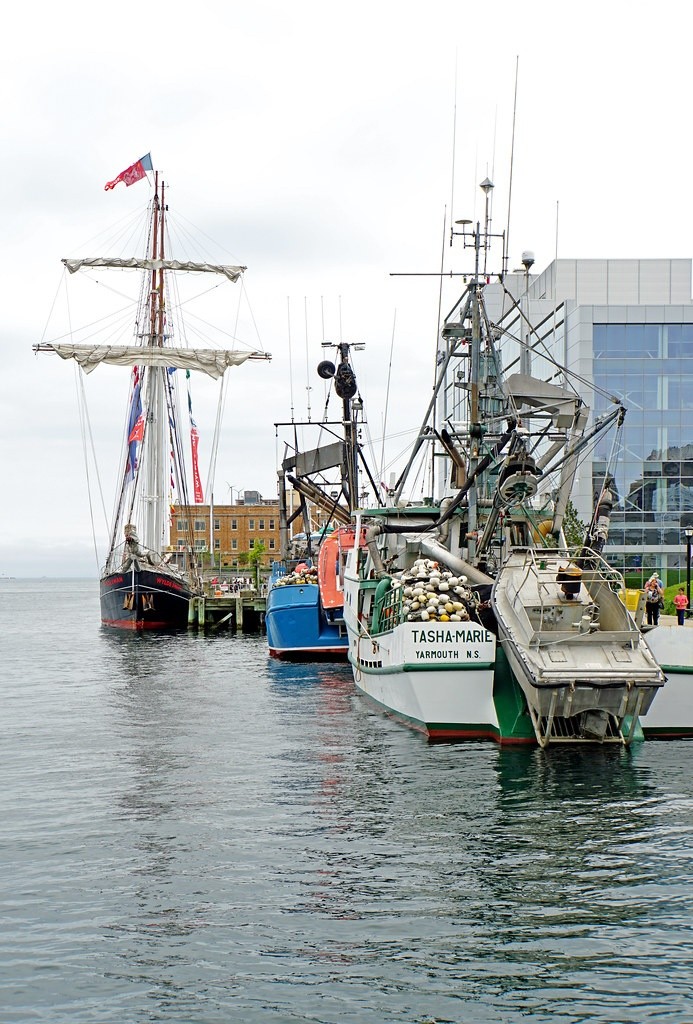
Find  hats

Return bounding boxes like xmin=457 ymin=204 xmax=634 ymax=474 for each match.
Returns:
xmin=652 ymin=573 xmax=659 ymax=578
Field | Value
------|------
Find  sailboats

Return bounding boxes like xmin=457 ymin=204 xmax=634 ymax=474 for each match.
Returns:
xmin=26 ymin=150 xmax=273 ymax=638
xmin=257 ymin=333 xmax=383 ymax=658
xmin=338 ymin=56 xmax=668 ymax=758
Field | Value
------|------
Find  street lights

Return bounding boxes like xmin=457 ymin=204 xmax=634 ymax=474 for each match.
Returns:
xmin=683 ymin=523 xmax=693 ymax=620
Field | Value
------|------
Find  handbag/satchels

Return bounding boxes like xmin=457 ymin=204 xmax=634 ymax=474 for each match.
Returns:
xmin=659 ymin=602 xmax=665 ymax=610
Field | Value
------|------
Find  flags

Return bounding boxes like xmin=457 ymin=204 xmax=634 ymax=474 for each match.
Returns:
xmin=123 ymin=385 xmax=143 ymax=486
xmin=120 ymin=153 xmax=153 ymax=187
xmin=191 ymin=428 xmax=204 ymax=503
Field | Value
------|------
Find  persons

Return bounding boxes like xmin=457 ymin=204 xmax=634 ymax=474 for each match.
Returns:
xmin=674 ymin=588 xmax=689 ymax=625
xmin=211 ymin=576 xmax=252 ymax=593
xmin=593 ymin=492 xmax=599 ymax=507
xmin=644 ymin=572 xmax=665 ymax=625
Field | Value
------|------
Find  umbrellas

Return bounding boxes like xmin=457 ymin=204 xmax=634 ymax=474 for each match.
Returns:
xmin=292 ymin=532 xmax=326 ymax=540
